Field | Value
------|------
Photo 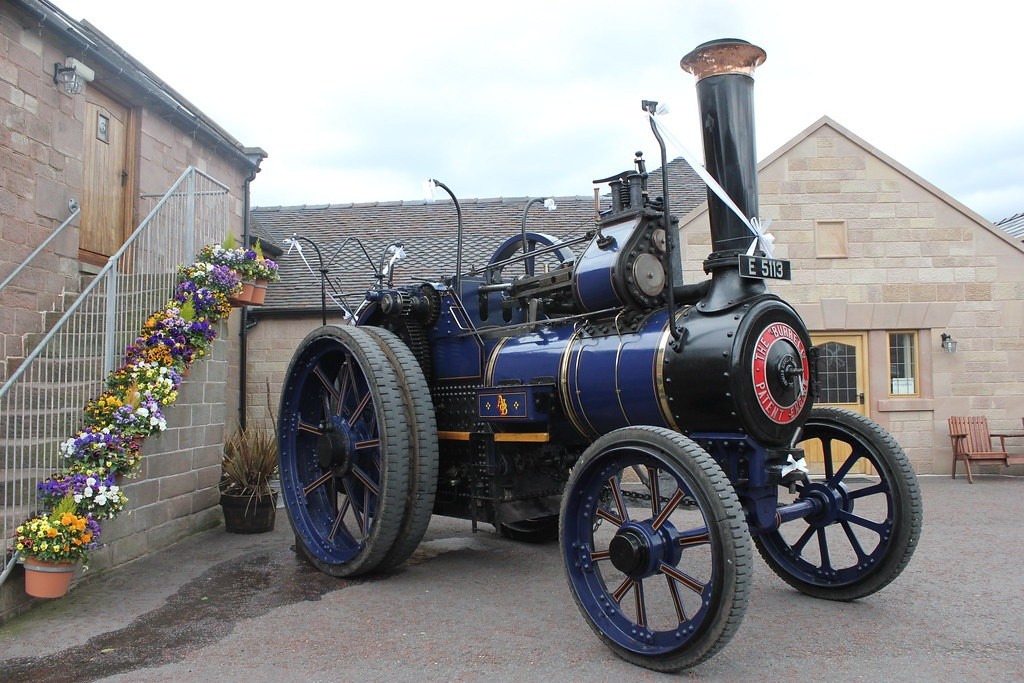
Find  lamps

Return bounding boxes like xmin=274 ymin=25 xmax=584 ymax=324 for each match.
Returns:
xmin=53 ymin=62 xmax=82 ymax=94
xmin=941 ymin=333 xmax=958 ymax=353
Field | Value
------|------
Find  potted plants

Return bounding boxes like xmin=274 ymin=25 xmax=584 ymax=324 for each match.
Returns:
xmin=210 ymin=415 xmax=278 ymax=533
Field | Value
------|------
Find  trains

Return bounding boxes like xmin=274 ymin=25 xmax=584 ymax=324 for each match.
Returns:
xmin=273 ymin=36 xmax=923 ymax=678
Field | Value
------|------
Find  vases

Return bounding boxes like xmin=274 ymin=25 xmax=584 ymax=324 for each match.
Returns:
xmin=246 ymin=280 xmax=268 ymax=305
xmin=23 ymin=556 xmax=76 ymax=598
xmin=233 ymin=280 xmax=256 ymax=303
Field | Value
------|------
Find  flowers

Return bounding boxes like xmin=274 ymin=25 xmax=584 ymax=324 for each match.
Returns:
xmin=15 ymin=246 xmax=280 ymax=570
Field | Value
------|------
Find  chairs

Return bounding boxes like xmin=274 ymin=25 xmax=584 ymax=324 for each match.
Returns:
xmin=948 ymin=415 xmax=1009 ymax=483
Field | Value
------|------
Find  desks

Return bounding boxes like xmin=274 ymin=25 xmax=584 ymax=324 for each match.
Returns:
xmin=990 ymin=433 xmax=1024 ymax=467
